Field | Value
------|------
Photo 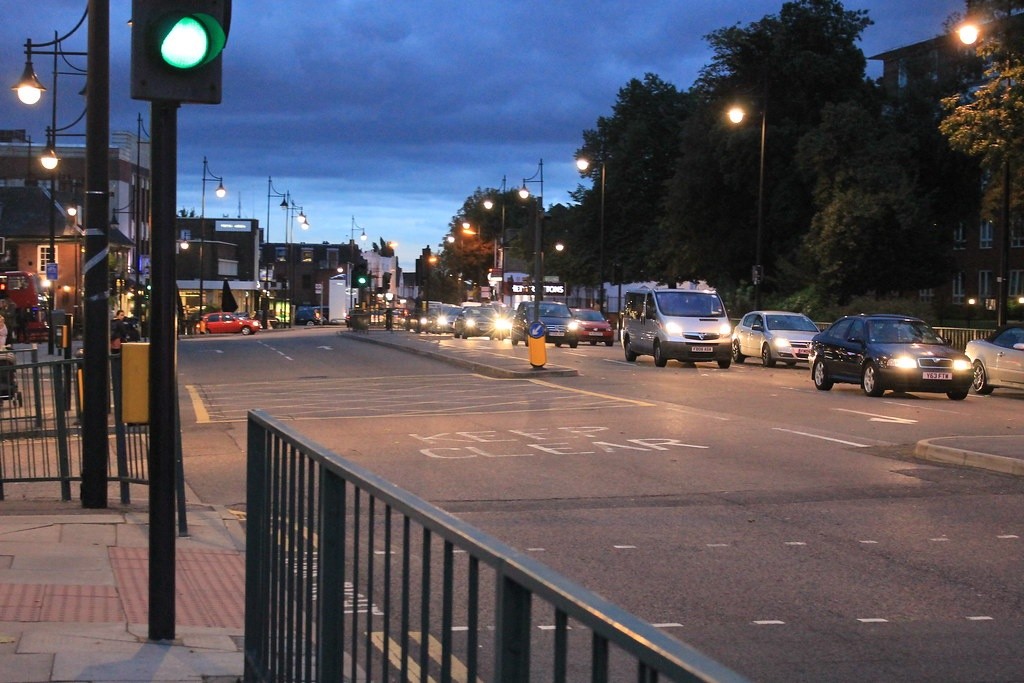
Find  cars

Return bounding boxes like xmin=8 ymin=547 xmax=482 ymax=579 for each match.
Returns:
xmin=406 ymin=305 xmax=510 ymax=341
xmin=806 ymin=313 xmax=974 ymax=401
xmin=569 ymin=307 xmax=614 ymax=346
xmin=964 ymin=327 xmax=1024 ymax=395
xmin=731 ymin=311 xmax=821 ymax=368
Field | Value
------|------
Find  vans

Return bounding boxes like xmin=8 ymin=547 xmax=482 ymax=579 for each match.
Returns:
xmin=620 ymin=288 xmax=732 ymax=370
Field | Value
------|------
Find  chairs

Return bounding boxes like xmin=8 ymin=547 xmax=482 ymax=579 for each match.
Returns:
xmin=848 ymin=327 xmax=899 ymax=338
xmin=0 ymin=352 xmax=24 ymax=408
xmin=751 ymin=325 xmax=761 ymax=330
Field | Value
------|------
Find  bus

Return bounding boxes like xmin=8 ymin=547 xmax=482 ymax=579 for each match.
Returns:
xmin=0 ymin=271 xmax=46 ymax=344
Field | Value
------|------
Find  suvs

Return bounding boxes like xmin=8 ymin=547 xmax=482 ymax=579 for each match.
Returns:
xmin=510 ymin=301 xmax=578 ymax=348
xmin=202 ymin=312 xmax=261 ymax=336
xmin=295 ymin=309 xmax=321 ymax=326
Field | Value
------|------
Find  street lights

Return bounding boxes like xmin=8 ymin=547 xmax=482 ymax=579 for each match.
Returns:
xmin=483 ymin=175 xmax=507 ymax=303
xmin=266 ymin=176 xmax=309 ymax=329
xmin=180 ymin=155 xmax=227 ymax=335
xmin=958 ymin=21 xmax=1007 ymax=328
xmin=576 ymin=158 xmax=605 ymax=313
xmin=518 ymin=157 xmax=544 ymax=301
xmin=427 ymin=209 xmax=481 ymax=305
xmin=11 ymin=2 xmax=112 ymax=508
xmin=725 ymin=106 xmax=767 ymax=311
xmin=350 ymin=214 xmax=367 ymax=310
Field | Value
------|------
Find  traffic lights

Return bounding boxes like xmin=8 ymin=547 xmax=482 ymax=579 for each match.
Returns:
xmin=356 ymin=265 xmax=367 ymax=287
xmin=131 ymin=0 xmax=232 ymax=105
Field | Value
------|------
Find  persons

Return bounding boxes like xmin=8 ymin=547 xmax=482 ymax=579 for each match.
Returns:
xmin=0 ymin=313 xmax=13 ymax=352
xmin=109 ymin=309 xmax=129 ymax=354
